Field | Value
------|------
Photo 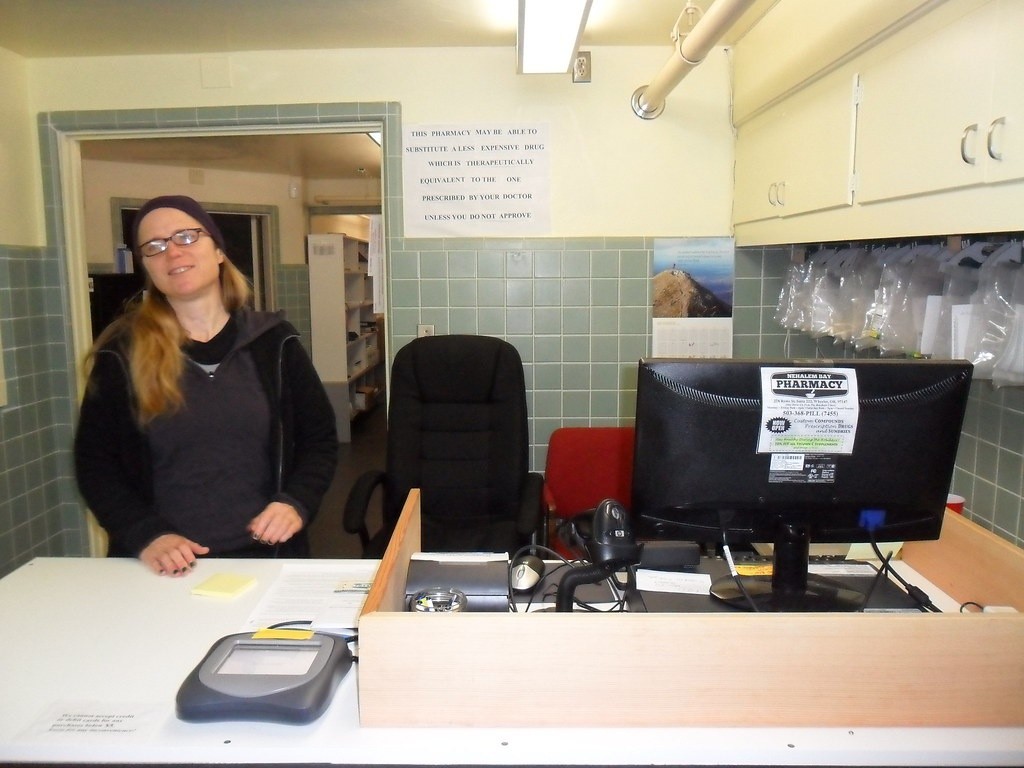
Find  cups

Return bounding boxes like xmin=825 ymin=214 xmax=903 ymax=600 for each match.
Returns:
xmin=411 ymin=587 xmax=467 ymax=612
xmin=947 ymin=494 xmax=964 ymax=514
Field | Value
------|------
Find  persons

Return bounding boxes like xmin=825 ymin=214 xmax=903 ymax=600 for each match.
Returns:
xmin=72 ymin=195 xmax=340 ymax=578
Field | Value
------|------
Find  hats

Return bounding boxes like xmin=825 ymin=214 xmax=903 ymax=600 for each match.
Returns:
xmin=133 ymin=194 xmax=226 ymax=254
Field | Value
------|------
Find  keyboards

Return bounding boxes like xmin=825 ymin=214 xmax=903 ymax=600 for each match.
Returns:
xmin=723 ymin=551 xmax=861 ymax=564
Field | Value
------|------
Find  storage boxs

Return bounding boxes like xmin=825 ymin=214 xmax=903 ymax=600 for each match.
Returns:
xmin=354 ymin=391 xmax=378 ymax=410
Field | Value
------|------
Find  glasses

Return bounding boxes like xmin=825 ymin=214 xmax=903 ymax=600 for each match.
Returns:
xmin=136 ymin=228 xmax=211 ymax=257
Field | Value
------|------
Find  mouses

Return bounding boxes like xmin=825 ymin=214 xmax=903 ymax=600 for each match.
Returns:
xmin=511 ymin=555 xmax=546 ymax=593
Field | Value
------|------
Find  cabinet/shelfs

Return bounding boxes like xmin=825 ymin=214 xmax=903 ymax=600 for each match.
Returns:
xmin=308 ymin=233 xmax=385 ymax=444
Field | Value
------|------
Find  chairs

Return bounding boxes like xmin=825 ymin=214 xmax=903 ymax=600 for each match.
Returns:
xmin=541 ymin=427 xmax=637 ymax=561
xmin=342 ymin=334 xmax=550 ymax=560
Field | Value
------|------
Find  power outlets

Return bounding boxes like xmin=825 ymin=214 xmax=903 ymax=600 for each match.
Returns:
xmin=573 ymin=51 xmax=591 ymax=82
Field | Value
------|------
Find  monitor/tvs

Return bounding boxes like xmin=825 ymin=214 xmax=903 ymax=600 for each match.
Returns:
xmin=624 ymin=360 xmax=974 ymax=611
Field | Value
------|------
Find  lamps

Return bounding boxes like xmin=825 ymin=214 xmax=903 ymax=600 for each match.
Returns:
xmin=514 ymin=0 xmax=593 ymax=75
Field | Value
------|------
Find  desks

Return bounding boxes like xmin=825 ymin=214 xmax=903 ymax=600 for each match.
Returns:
xmin=0 ymin=505 xmax=1024 ymax=768
